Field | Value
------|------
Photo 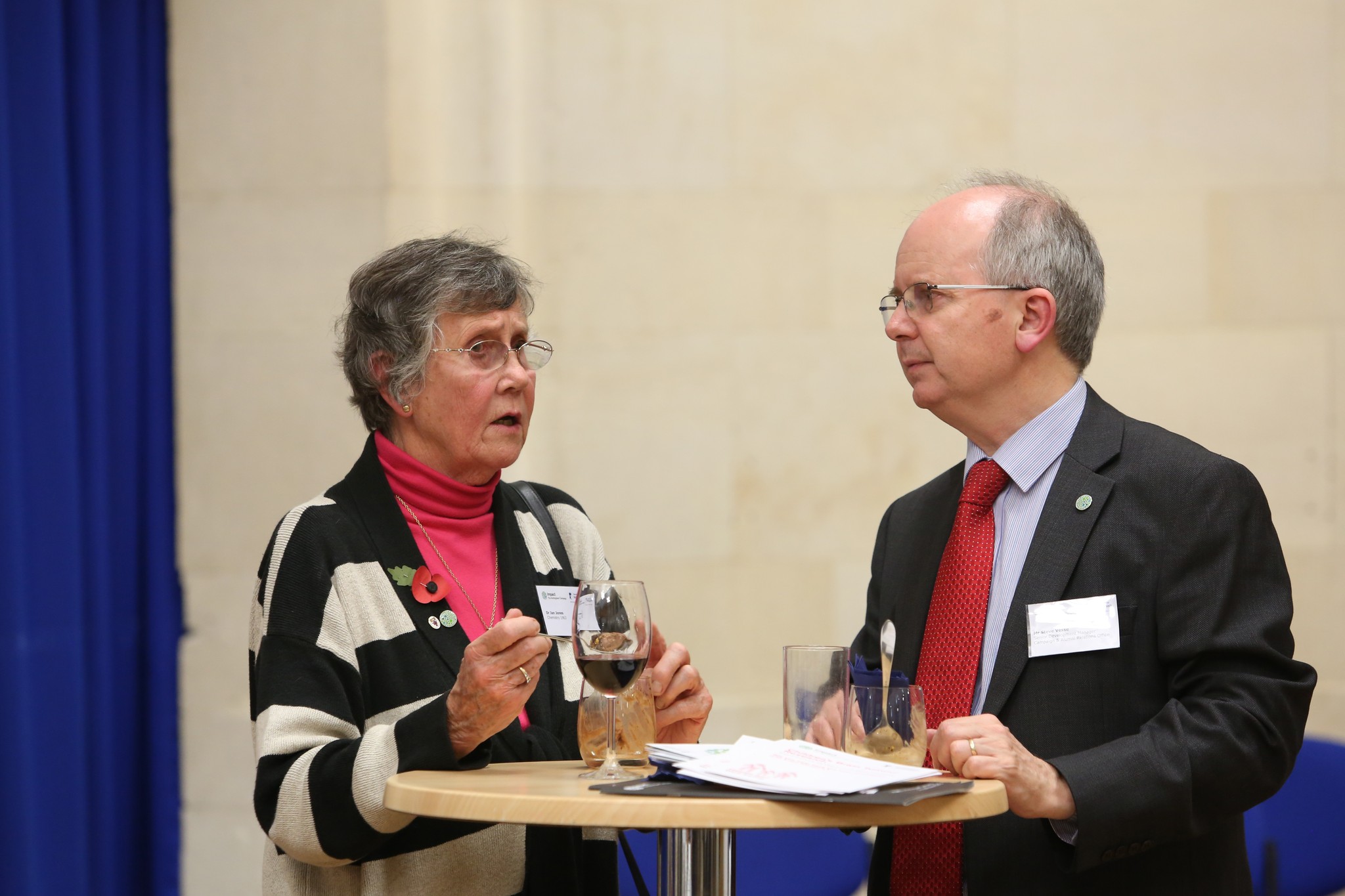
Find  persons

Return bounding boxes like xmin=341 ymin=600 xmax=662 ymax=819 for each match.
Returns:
xmin=249 ymin=233 xmax=714 ymax=896
xmin=801 ymin=178 xmax=1320 ymax=896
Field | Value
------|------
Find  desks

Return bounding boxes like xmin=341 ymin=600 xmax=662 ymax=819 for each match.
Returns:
xmin=382 ymin=759 xmax=1009 ymax=896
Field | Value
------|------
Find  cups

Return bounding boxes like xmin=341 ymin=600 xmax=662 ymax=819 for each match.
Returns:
xmin=783 ymin=645 xmax=850 ymax=753
xmin=578 ymin=673 xmax=656 ymax=770
xmin=840 ymin=683 xmax=927 ymax=768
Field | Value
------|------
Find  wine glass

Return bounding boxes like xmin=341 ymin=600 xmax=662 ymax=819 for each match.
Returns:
xmin=571 ymin=580 xmax=652 ymax=781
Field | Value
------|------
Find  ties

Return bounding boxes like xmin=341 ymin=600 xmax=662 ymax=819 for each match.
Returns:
xmin=889 ymin=460 xmax=1012 ymax=896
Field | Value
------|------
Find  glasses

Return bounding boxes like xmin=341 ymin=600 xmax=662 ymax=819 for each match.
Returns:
xmin=879 ymin=282 xmax=1030 ymax=327
xmin=430 ymin=340 xmax=554 ymax=370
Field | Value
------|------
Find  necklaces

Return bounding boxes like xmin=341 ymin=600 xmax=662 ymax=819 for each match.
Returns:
xmin=395 ymin=495 xmax=498 ymax=631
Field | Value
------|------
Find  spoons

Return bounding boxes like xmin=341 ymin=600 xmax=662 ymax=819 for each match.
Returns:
xmin=501 ymin=618 xmax=633 ymax=654
xmin=863 ymin=619 xmax=903 ymax=755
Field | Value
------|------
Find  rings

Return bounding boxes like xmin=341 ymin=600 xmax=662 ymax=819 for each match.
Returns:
xmin=518 ymin=666 xmax=532 ymax=685
xmin=968 ymin=738 xmax=976 ymax=755
